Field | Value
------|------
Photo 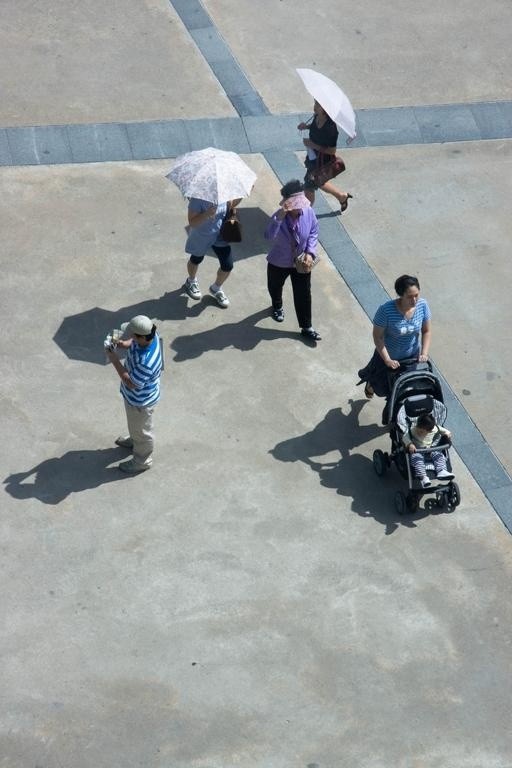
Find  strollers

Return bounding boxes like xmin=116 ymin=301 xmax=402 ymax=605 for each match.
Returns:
xmin=374 ymin=357 xmax=459 ymax=515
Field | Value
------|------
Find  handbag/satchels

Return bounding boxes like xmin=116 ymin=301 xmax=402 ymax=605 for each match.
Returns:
xmin=223 ymin=207 xmax=241 ymax=242
xmin=159 ymin=337 xmax=165 ymax=370
xmin=311 ymin=157 xmax=345 ymax=185
xmin=296 ymin=250 xmax=321 ymax=273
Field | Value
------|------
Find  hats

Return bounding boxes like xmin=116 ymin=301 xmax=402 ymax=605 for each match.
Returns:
xmin=279 ymin=190 xmax=311 ymax=210
xmin=121 ymin=314 xmax=153 ymax=335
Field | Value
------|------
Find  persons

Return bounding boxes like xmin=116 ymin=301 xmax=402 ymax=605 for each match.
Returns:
xmin=103 ymin=313 xmax=164 ymax=475
xmin=262 ymin=179 xmax=324 ymax=343
xmin=355 ymin=274 xmax=430 ymax=399
xmin=401 ymin=412 xmax=455 ymax=486
xmin=298 ymin=100 xmax=353 ymax=213
xmin=182 ymin=184 xmax=256 ymax=309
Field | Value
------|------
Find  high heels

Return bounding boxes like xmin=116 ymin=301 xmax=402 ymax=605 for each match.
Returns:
xmin=340 ymin=192 xmax=353 ymax=210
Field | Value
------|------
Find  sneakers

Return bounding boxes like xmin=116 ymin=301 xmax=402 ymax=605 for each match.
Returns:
xmin=365 ymin=381 xmax=374 ymax=397
xmin=423 ymin=475 xmax=432 ymax=486
xmin=119 ymin=456 xmax=150 ymax=472
xmin=184 ymin=277 xmax=201 ymax=299
xmin=272 ymin=308 xmax=284 ymax=321
xmin=301 ymin=327 xmax=322 ymax=340
xmin=437 ymin=469 xmax=455 ymax=480
xmin=117 ymin=435 xmax=133 ymax=447
xmin=209 ymin=285 xmax=229 ymax=307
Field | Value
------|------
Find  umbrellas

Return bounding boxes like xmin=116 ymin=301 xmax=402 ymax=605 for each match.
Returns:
xmin=164 ymin=145 xmax=259 ymax=206
xmin=296 ymin=67 xmax=357 ymax=138
